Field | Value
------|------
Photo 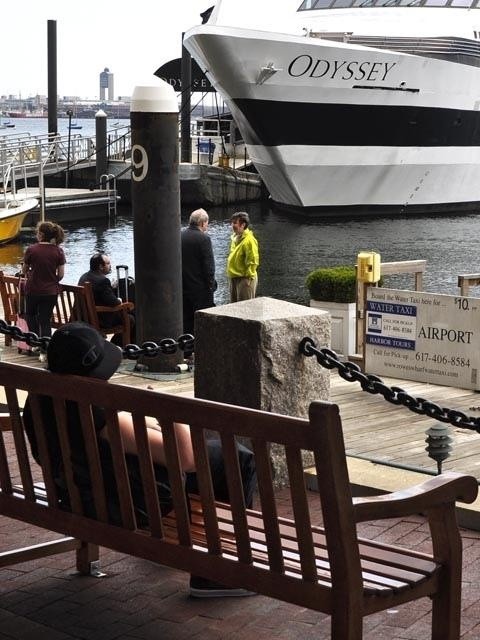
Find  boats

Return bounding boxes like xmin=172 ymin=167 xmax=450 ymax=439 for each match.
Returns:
xmin=67 ymin=123 xmax=83 ymax=129
xmin=111 ymin=122 xmax=124 ymax=128
xmin=184 ymin=0 xmax=480 ymax=222
xmin=1 ymin=195 xmax=38 ymax=248
xmin=0 ymin=240 xmax=26 ymax=270
xmin=0 ymin=119 xmax=15 ymax=128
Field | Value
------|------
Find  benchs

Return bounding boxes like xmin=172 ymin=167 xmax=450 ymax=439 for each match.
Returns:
xmin=0 ymin=358 xmax=480 ymax=640
xmin=0 ymin=267 xmax=137 ymax=358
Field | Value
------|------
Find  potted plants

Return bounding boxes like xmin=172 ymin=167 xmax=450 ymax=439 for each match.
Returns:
xmin=304 ymin=265 xmax=383 ymax=358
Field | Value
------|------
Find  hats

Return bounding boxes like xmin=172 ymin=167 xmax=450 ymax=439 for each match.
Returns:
xmin=45 ymin=322 xmax=123 ymax=385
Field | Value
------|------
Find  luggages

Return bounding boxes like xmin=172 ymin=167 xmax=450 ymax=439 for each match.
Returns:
xmin=14 ymin=271 xmax=41 ymax=354
xmin=115 ymin=264 xmax=135 ymax=307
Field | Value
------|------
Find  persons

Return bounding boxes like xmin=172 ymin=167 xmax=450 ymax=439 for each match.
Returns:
xmin=225 ymin=212 xmax=261 ymax=302
xmin=73 ymin=254 xmax=135 ymax=348
xmin=13 ymin=245 xmax=30 ymax=315
xmin=182 ymin=206 xmax=219 ymax=360
xmin=25 ymin=319 xmax=260 ymax=600
xmin=22 ymin=220 xmax=68 ymax=361
